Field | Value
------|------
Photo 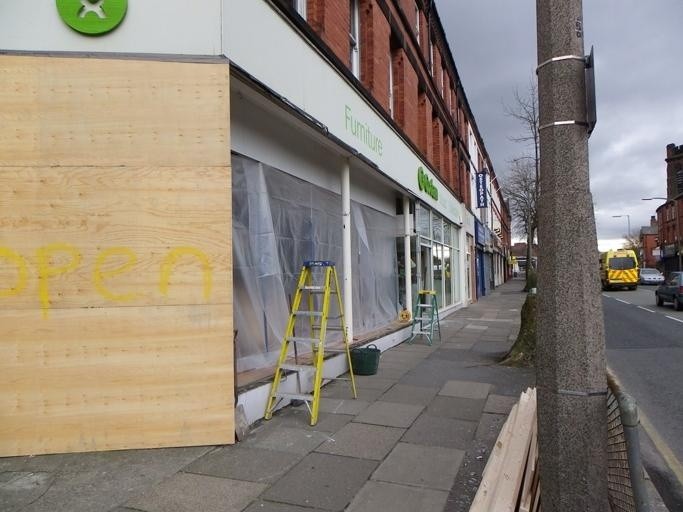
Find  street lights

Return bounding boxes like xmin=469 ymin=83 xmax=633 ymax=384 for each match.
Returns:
xmin=642 ymin=197 xmax=681 ymax=271
xmin=611 ymin=214 xmax=630 ymax=239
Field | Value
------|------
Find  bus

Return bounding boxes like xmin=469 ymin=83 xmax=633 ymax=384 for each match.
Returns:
xmin=599 ymin=249 xmax=640 ymax=290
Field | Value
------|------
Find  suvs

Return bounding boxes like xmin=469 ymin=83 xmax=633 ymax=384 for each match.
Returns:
xmin=655 ymin=270 xmax=683 ymax=310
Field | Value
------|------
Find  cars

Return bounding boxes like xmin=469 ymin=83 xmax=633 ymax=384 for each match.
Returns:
xmin=639 ymin=267 xmax=665 ymax=285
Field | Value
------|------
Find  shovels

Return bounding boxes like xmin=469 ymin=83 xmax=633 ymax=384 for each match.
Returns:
xmin=234 ymin=328 xmax=248 ymax=443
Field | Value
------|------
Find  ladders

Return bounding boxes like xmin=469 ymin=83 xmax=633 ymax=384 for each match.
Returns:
xmin=265 ymin=262 xmax=357 ymax=426
xmin=409 ymin=290 xmax=442 ymax=346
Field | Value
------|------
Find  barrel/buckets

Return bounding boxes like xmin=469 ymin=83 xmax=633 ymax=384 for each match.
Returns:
xmin=350 ymin=343 xmax=382 ymax=375
